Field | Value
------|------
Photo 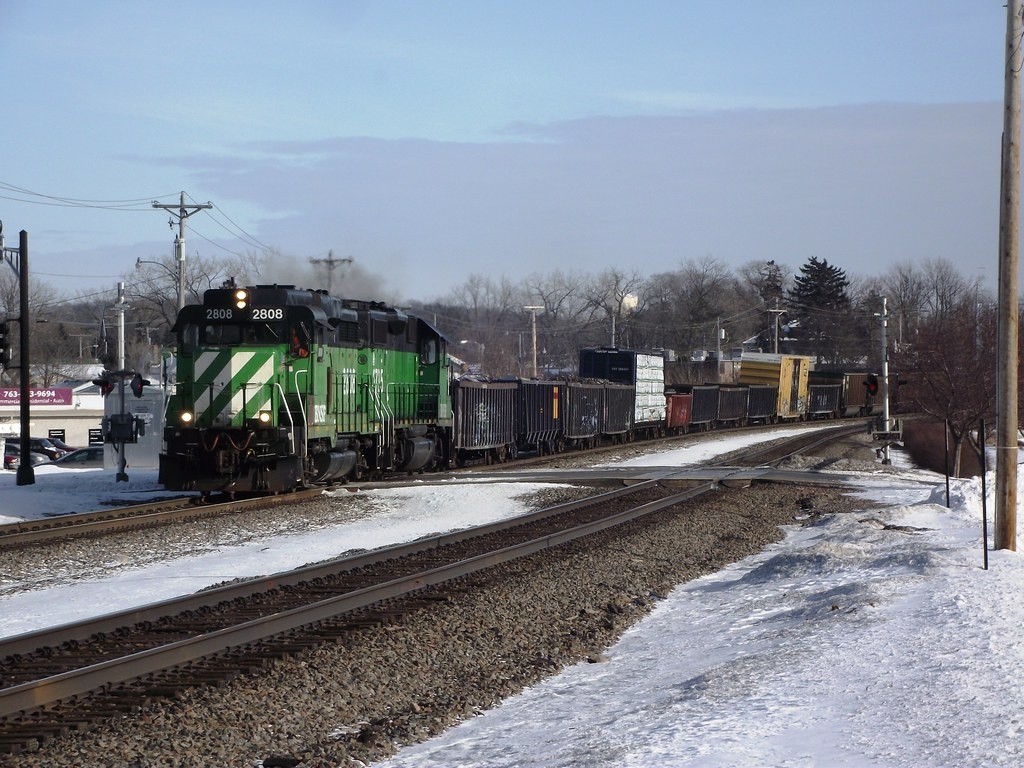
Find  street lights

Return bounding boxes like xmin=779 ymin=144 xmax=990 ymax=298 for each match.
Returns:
xmin=135 ymin=257 xmax=184 ymax=313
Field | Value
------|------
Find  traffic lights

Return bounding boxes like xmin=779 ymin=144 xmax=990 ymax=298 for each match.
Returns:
xmin=123 ymin=370 xmax=150 ymax=399
xmin=92 ymin=372 xmax=119 ymax=397
xmin=0 ymin=321 xmax=9 ymax=369
xmin=863 ymin=373 xmax=878 ymax=397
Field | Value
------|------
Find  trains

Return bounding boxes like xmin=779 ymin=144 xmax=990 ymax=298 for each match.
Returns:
xmin=163 ymin=284 xmax=948 ymax=503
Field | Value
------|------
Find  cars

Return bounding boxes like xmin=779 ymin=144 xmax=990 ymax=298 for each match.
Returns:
xmin=2 ymin=437 xmax=104 ymax=468
